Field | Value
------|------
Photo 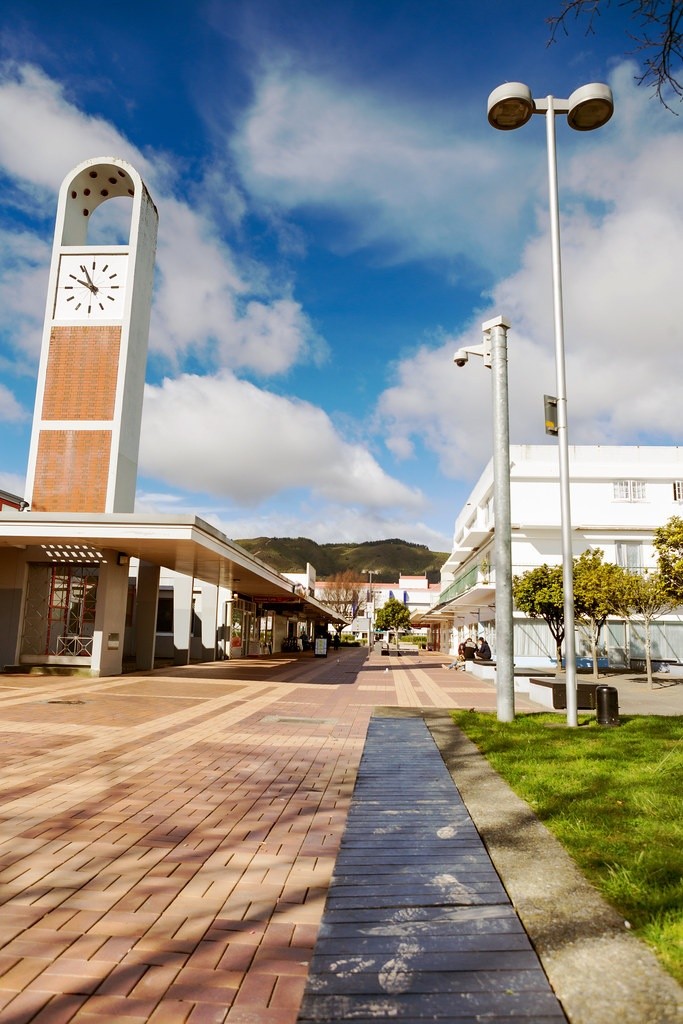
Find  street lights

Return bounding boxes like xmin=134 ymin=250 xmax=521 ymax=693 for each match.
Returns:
xmin=486 ymin=81 xmax=615 ymax=729
xmin=452 ymin=314 xmax=516 ymax=724
xmin=362 ymin=570 xmax=382 ymax=657
xmin=369 ymin=589 xmax=384 ymax=650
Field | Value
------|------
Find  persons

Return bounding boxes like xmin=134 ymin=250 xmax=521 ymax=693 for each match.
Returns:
xmin=300 ymin=630 xmax=341 ymax=651
xmin=442 ymin=637 xmax=491 ymax=672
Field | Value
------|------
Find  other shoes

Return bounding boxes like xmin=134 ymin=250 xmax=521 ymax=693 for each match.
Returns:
xmin=441 ymin=663 xmax=450 ymax=670
